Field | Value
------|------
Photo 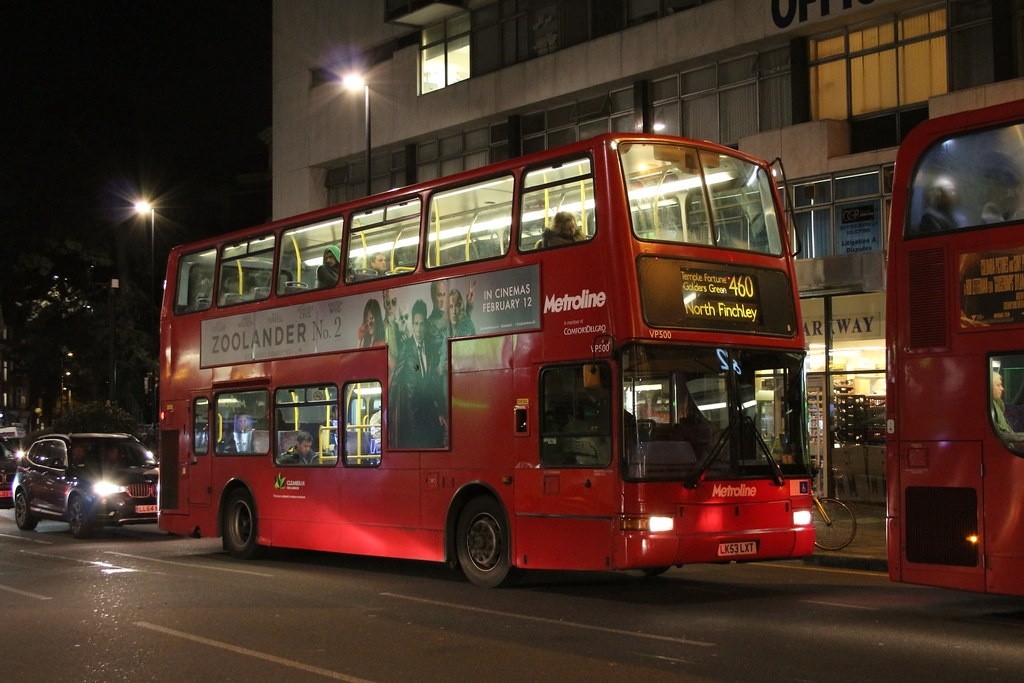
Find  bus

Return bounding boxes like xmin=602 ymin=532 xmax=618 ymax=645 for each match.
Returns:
xmin=155 ymin=134 xmax=817 ymax=588
xmin=886 ymin=99 xmax=1024 ymax=596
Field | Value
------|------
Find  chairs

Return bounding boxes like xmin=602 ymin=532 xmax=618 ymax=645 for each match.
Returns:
xmin=277 ymin=430 xmax=303 ymax=454
xmin=198 ymin=267 xmax=378 ymax=309
xmin=251 ymin=430 xmax=269 ymax=452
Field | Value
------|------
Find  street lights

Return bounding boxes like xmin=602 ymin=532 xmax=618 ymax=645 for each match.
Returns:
xmin=132 ymin=198 xmax=156 ymax=432
xmin=336 ymin=66 xmax=372 ymax=197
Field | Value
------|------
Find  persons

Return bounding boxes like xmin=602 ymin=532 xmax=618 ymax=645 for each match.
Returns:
xmin=918 ymin=178 xmax=959 ymax=233
xmin=541 ymin=212 xmax=586 ymax=248
xmin=991 ymin=371 xmax=1024 ymax=450
xmin=317 ymin=246 xmax=389 ymax=290
xmin=358 ymin=279 xmax=477 ymax=448
xmin=218 ymin=405 xmax=381 ymax=464
xmin=69 ymin=444 xmax=132 ymax=466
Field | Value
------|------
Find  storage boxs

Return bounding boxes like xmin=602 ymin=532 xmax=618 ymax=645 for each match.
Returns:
xmin=831 ymin=395 xmax=887 ymax=503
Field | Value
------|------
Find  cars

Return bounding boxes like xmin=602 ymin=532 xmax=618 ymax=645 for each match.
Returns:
xmin=13 ymin=433 xmax=160 ymax=538
xmin=0 ymin=436 xmax=26 ymax=507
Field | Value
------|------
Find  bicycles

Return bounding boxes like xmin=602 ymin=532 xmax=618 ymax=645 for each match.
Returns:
xmin=810 ymin=463 xmax=856 ymax=551
